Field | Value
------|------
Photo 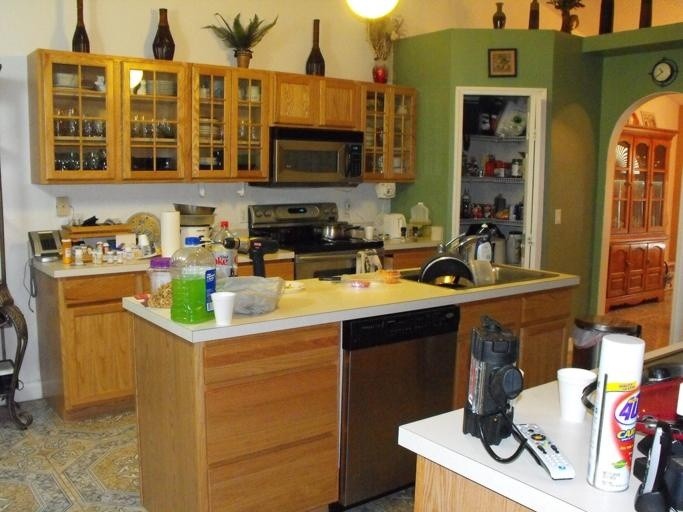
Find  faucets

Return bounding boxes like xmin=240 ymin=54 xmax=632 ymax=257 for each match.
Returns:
xmin=452 ymin=234 xmax=490 ymax=256
xmin=438 ymin=232 xmax=466 ymax=255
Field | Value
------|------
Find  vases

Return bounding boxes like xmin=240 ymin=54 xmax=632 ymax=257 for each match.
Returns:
xmin=527 ymin=0 xmax=651 ymax=36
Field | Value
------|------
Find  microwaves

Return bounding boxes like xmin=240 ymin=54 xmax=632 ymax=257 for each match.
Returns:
xmin=273 ymin=136 xmax=362 ymax=187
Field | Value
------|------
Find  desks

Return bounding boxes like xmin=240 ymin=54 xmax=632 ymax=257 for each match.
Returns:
xmin=395 ymin=368 xmax=682 ymax=512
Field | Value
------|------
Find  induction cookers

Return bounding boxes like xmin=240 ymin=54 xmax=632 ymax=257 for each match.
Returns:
xmin=254 ymin=226 xmax=384 ymax=253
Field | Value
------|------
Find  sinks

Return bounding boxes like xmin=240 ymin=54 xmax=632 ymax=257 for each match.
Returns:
xmin=398 ymin=262 xmax=562 ymax=291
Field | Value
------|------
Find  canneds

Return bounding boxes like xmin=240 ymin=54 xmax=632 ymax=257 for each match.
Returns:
xmin=512 ymin=159 xmax=523 ymax=178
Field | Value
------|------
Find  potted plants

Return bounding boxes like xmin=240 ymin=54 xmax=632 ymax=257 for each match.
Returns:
xmin=367 ymin=14 xmax=408 ymax=84
xmin=199 ymin=10 xmax=280 ymax=69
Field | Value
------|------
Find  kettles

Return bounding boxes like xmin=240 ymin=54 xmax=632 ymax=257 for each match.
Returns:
xmin=383 ymin=212 xmax=408 ymax=239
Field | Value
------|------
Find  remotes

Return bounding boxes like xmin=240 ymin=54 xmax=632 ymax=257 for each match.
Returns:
xmin=511 ymin=423 xmax=576 ymax=480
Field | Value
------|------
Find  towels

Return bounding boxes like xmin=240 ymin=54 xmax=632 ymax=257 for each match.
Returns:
xmin=355 ymin=248 xmax=386 ymax=275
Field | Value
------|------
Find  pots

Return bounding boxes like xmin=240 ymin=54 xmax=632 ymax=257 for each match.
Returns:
xmin=320 ymin=215 xmax=360 ymax=239
xmin=418 ymin=252 xmax=474 ymax=288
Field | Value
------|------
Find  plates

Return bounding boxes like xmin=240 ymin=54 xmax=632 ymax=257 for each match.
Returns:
xmin=280 ymin=280 xmax=305 ymax=294
xmin=199 ymin=124 xmax=219 ymax=138
xmin=144 ymin=80 xmax=174 ymax=97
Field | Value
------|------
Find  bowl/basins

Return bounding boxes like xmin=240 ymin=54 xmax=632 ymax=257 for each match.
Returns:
xmin=198 ymin=150 xmax=223 ymax=170
xmin=237 ymin=163 xmax=256 ymax=170
xmin=134 ymin=157 xmax=173 ymax=170
xmin=467 ymin=257 xmax=495 ymax=286
xmin=54 ymin=73 xmax=81 ymax=86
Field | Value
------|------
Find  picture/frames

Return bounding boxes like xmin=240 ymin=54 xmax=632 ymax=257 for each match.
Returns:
xmin=487 ymin=48 xmax=517 ymax=77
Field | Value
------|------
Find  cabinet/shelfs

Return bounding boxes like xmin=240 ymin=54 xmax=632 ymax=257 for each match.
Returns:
xmin=390 ymin=245 xmax=446 ymax=270
xmin=610 ymin=125 xmax=649 ymax=233
xmin=272 ymin=72 xmax=359 ymax=130
xmin=129 ymin=312 xmax=339 ymax=512
xmin=29 ymin=269 xmax=144 ymax=411
xmin=521 ymin=286 xmax=573 ymax=390
xmin=454 ymin=293 xmax=521 ymax=413
xmin=359 ymin=80 xmax=417 ymax=182
xmin=234 ymin=255 xmax=298 ymax=281
xmin=118 ymin=58 xmax=190 ymax=184
xmin=649 ymin=128 xmax=678 ymax=233
xmin=0 ymin=278 xmax=34 ymax=431
xmin=604 ymin=233 xmax=668 ymax=313
xmin=461 ymin=132 xmax=528 ymax=270
xmin=26 ymin=46 xmax=118 ymax=185
xmin=190 ymin=62 xmax=272 ymax=180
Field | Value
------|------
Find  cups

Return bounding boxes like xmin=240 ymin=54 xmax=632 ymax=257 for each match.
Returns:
xmin=237 ymin=120 xmax=257 ymax=141
xmin=54 ymin=148 xmax=107 ymax=171
xmin=209 ymin=290 xmax=235 ymax=325
xmin=553 ymin=365 xmax=597 ymax=425
xmin=51 ymin=105 xmax=173 ymax=137
xmin=363 ymin=227 xmax=374 ymax=240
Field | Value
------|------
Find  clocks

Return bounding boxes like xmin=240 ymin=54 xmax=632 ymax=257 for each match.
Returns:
xmin=646 ymin=54 xmax=680 ymax=88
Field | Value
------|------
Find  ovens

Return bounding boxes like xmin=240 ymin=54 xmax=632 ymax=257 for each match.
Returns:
xmin=295 ymin=253 xmax=372 ymax=279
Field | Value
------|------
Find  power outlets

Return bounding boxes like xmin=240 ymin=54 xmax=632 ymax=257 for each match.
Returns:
xmin=56 ymin=197 xmax=70 ymax=217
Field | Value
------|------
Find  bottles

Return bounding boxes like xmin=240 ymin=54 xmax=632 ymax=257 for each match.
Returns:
xmin=510 ymin=158 xmax=523 ymax=177
xmin=460 ymin=184 xmax=472 ymax=217
xmin=527 ymin=0 xmax=540 ymax=30
xmin=597 ymin=0 xmax=651 ymax=36
xmin=212 ymin=219 xmax=239 ymax=277
xmin=588 ymin=332 xmax=644 ymax=493
xmin=60 ymin=233 xmax=151 ymax=265
xmin=474 ymin=223 xmax=494 ymax=263
